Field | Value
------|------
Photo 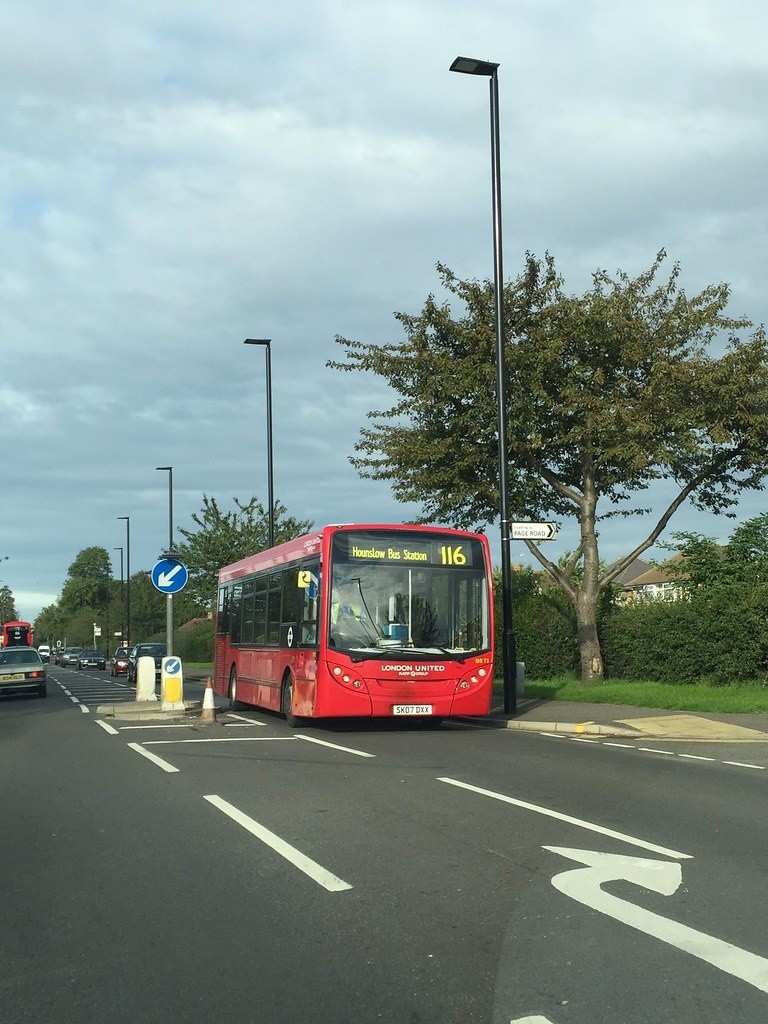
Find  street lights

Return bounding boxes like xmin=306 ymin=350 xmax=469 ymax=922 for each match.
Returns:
xmin=117 ymin=516 xmax=131 ymax=647
xmin=242 ymin=337 xmax=277 ymax=548
xmin=450 ymin=54 xmax=521 ymax=718
xmin=106 ymin=570 xmax=114 ymax=661
xmin=155 ymin=466 xmax=175 ymax=554
xmin=113 ymin=546 xmax=124 ymax=646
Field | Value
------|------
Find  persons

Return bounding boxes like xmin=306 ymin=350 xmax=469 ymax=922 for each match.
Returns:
xmin=118 ymin=651 xmax=126 ymax=657
xmin=331 ymin=588 xmax=366 ymax=623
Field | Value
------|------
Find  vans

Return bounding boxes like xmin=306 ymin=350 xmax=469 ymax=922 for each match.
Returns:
xmin=37 ymin=645 xmax=51 ymax=661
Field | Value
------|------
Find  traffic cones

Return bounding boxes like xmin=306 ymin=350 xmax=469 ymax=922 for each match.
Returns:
xmin=191 ymin=676 xmax=224 ymax=726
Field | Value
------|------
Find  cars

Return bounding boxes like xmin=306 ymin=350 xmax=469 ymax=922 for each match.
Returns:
xmin=54 ymin=647 xmax=107 ymax=671
xmin=109 ymin=647 xmax=134 ymax=677
xmin=0 ymin=647 xmax=50 ymax=698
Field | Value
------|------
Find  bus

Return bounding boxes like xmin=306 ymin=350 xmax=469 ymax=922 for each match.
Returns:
xmin=1 ymin=620 xmax=35 ymax=648
xmin=210 ymin=522 xmax=497 ymax=729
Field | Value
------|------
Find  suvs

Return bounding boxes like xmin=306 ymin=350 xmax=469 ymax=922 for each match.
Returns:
xmin=126 ymin=642 xmax=167 ymax=686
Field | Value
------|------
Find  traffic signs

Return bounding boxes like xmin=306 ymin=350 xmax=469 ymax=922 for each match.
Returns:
xmin=510 ymin=522 xmax=559 ymax=541
